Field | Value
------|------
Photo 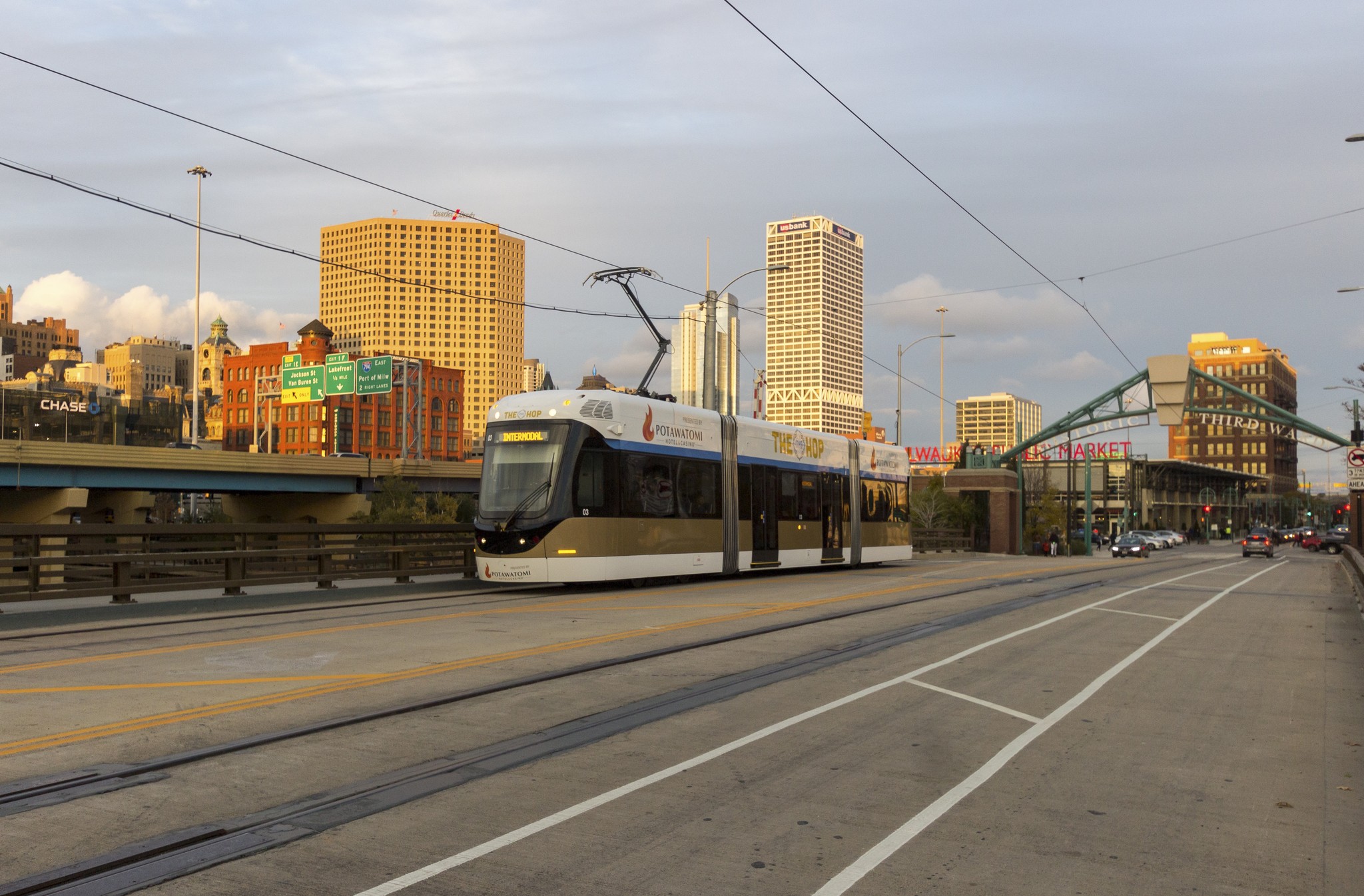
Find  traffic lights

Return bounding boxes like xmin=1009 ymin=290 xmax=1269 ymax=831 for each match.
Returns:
xmin=1204 ymin=505 xmax=1210 ymax=512
xmin=1307 ymin=512 xmax=1311 ymax=517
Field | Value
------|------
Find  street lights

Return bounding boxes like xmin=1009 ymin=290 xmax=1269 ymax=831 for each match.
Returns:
xmin=1243 ymin=492 xmax=1258 ymax=534
xmin=1222 ymin=487 xmax=1239 ymax=543
xmin=896 ymin=334 xmax=956 ymax=449
xmin=1199 ymin=487 xmax=1216 ymax=545
xmin=697 ymin=265 xmax=791 ymax=413
xmin=1259 ymin=494 xmax=1273 ymax=527
xmin=186 ymin=165 xmax=212 ymax=450
xmin=936 ymin=307 xmax=948 ymax=468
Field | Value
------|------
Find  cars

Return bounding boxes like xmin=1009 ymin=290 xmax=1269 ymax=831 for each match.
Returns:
xmin=1242 ymin=533 xmax=1274 ymax=558
xmin=1070 ymin=529 xmax=1110 ymax=545
xmin=329 ymin=453 xmax=368 ymax=458
xmin=1251 ymin=524 xmax=1351 ymax=554
xmin=1112 ymin=536 xmax=1149 ymax=559
xmin=1114 ymin=534 xmax=1165 ymax=551
xmin=1155 ymin=530 xmax=1183 ymax=546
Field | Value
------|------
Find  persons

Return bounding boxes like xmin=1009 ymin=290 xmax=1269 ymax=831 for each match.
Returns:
xmin=1031 ymin=530 xmax=1041 ymax=556
xmin=1219 ymin=528 xmax=1225 ymax=540
xmin=1298 ymin=531 xmax=1303 ymax=546
xmin=1107 ymin=531 xmax=1117 ymax=552
xmin=1096 ymin=533 xmax=1103 ymax=551
xmin=1196 ymin=529 xmax=1201 ymax=544
xmin=1049 ymin=531 xmax=1059 ymax=557
xmin=1041 ymin=539 xmax=1050 ymax=557
xmin=1292 ymin=531 xmax=1299 ymax=547
xmin=1184 ymin=529 xmax=1191 ymax=545
xmin=1225 ymin=526 xmax=1231 ymax=540
xmin=1274 ymin=529 xmax=1280 ymax=546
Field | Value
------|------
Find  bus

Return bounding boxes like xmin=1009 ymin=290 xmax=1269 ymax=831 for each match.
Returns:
xmin=471 ymin=265 xmax=912 ymax=585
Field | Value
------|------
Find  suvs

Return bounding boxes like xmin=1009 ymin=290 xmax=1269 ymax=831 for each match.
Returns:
xmin=1129 ymin=530 xmax=1174 ymax=548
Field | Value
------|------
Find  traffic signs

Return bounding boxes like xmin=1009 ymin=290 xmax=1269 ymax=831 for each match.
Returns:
xmin=324 ymin=361 xmax=355 ymax=396
xmin=281 ymin=365 xmax=325 ymax=404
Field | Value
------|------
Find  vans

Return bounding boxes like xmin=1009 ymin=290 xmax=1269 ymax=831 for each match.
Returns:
xmin=166 ymin=443 xmax=202 ymax=449
